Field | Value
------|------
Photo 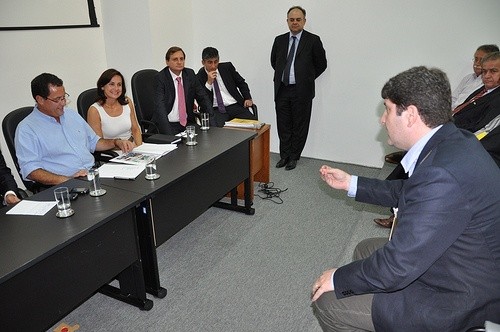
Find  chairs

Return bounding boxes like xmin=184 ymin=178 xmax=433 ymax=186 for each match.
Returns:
xmin=131 ymin=69 xmax=201 ymax=133
xmin=77 ymin=89 xmax=156 ymax=162
xmin=2 ymin=107 xmax=119 ymax=195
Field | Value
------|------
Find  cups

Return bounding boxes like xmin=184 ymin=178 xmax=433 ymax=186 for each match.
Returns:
xmin=54 ymin=187 xmax=71 ymax=217
xmin=87 ymin=170 xmax=100 ymax=195
xmin=144 ymin=154 xmax=157 ymax=177
xmin=201 ymin=113 xmax=210 ymax=129
xmin=186 ymin=126 xmax=195 ymax=144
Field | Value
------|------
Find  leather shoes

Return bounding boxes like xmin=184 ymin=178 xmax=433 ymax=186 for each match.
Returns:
xmin=374 ymin=218 xmax=392 ymax=228
xmin=384 ymin=150 xmax=406 ymax=165
xmin=285 ymin=159 xmax=297 ymax=170
xmin=276 ymin=159 xmax=287 ymax=168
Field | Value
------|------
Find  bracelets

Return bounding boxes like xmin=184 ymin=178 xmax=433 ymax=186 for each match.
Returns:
xmin=115 ymin=138 xmax=122 ymax=150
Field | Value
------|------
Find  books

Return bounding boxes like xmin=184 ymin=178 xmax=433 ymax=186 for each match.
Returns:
xmin=147 ymin=133 xmax=181 ymax=144
xmin=223 ymin=118 xmax=265 ymax=130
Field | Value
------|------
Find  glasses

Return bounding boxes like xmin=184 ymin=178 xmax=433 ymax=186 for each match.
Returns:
xmin=472 ymin=58 xmax=481 ymax=62
xmin=45 ymin=92 xmax=70 ymax=103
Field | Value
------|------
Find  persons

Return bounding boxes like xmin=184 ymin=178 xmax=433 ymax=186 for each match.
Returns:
xmin=271 ymin=6 xmax=327 ymax=170
xmin=374 ymin=45 xmax=500 ymax=229
xmin=0 ymin=148 xmax=21 ymax=208
xmin=311 ymin=66 xmax=500 ymax=332
xmin=196 ymin=47 xmax=255 ymax=127
xmin=148 ymin=46 xmax=218 ymax=144
xmin=87 ymin=69 xmax=142 ymax=167
xmin=14 ymin=73 xmax=134 ymax=194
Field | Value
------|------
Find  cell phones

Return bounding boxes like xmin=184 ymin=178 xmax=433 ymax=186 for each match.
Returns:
xmin=58 ymin=194 xmax=78 ymax=200
xmin=70 ymin=187 xmax=89 ymax=194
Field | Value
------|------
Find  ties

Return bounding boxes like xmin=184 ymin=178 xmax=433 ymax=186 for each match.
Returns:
xmin=283 ymin=36 xmax=297 ymax=87
xmin=176 ymin=77 xmax=187 ymax=127
xmin=453 ymin=89 xmax=487 ymax=114
xmin=213 ymin=77 xmax=226 ymax=113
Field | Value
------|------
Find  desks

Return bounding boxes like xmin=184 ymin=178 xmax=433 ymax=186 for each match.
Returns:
xmin=76 ymin=126 xmax=258 ymax=299
xmin=0 ymin=177 xmax=154 ymax=332
xmin=222 ymin=123 xmax=271 ymax=200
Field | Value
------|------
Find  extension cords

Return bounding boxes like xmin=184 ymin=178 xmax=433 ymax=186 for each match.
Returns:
xmin=258 ymin=186 xmax=278 ymax=191
